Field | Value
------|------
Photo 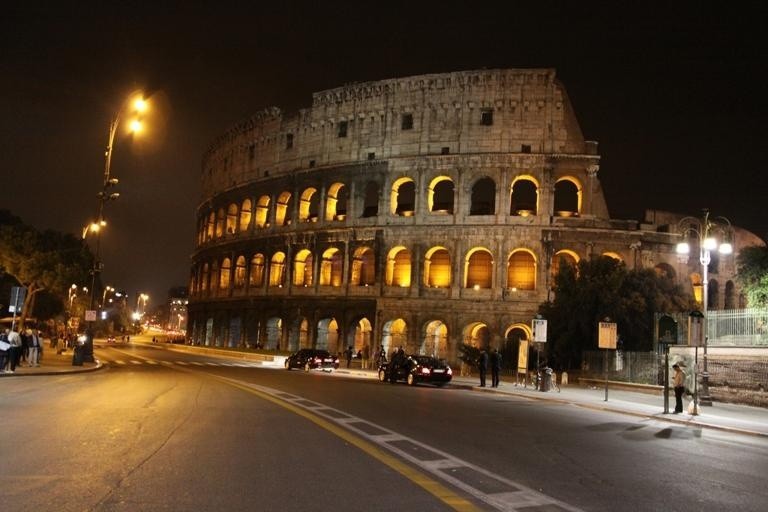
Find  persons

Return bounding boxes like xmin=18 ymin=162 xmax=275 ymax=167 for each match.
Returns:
xmin=0 ymin=325 xmax=44 ymax=376
xmin=275 ymin=340 xmax=280 ymax=353
xmin=399 ymin=344 xmax=405 ymax=357
xmin=390 ymin=346 xmax=400 ymax=367
xmin=670 ymin=363 xmax=686 ymax=416
xmin=120 ymin=334 xmax=125 ymax=344
xmin=126 ymin=335 xmax=130 ymax=342
xmin=151 ymin=335 xmax=155 ymax=345
xmin=377 ymin=344 xmax=387 ymax=367
xmin=472 ymin=346 xmax=487 ymax=388
xmin=372 ymin=346 xmax=380 ymax=363
xmin=356 ymin=350 xmax=361 ymax=358
xmin=344 ymin=346 xmax=351 ymax=368
xmin=360 ymin=349 xmax=371 ymax=370
xmin=489 ymin=347 xmax=503 ymax=388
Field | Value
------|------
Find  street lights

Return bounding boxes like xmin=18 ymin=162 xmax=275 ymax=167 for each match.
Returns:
xmin=657 ymin=330 xmax=674 ymax=416
xmin=136 ymin=293 xmax=149 ymax=318
xmin=67 ymin=284 xmax=150 ymax=318
xmin=676 ymin=207 xmax=734 ymax=406
xmin=177 ymin=314 xmax=183 ymax=329
xmin=82 ymin=84 xmax=161 ymax=363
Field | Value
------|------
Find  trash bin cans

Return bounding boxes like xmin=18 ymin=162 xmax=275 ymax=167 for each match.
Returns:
xmin=540 ymin=367 xmax=553 ymax=391
xmin=72 ymin=345 xmax=84 ymax=366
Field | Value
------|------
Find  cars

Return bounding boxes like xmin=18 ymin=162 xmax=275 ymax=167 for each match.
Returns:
xmin=284 ymin=347 xmax=341 ymax=372
xmin=376 ymin=352 xmax=455 ymax=387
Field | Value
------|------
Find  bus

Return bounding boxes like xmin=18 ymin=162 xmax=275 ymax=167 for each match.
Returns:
xmin=0 ymin=317 xmax=41 ymax=339
